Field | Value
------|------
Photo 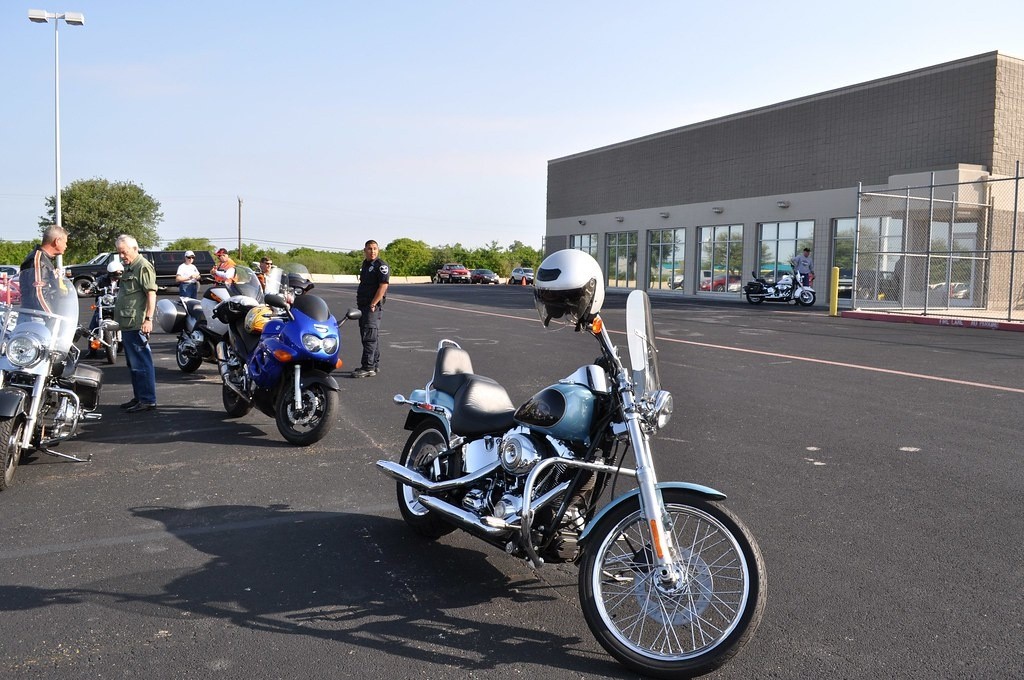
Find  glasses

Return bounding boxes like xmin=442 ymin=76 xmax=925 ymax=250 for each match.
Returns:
xmin=186 ymin=256 xmax=195 ymax=259
xmin=218 ymin=255 xmax=225 ymax=257
xmin=262 ymin=262 xmax=272 ymax=265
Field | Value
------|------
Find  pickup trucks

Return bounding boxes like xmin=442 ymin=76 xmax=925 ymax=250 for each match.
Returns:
xmin=437 ymin=263 xmax=472 ymax=284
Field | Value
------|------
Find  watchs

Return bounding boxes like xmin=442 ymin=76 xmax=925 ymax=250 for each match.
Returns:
xmin=145 ymin=317 xmax=152 ymax=321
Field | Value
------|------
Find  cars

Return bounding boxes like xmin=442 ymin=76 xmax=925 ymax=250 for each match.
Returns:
xmin=468 ymin=269 xmax=500 ymax=284
xmin=701 ymin=269 xmax=793 ymax=293
xmin=510 ymin=268 xmax=534 ymax=284
xmin=928 ymin=282 xmax=969 ymax=299
xmin=0 ymin=264 xmax=23 ymax=284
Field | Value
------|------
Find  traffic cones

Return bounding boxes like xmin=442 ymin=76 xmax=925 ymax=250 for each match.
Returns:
xmin=521 ymin=277 xmax=527 ymax=286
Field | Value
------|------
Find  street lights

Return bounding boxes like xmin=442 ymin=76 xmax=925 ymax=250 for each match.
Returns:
xmin=27 ymin=6 xmax=86 ymax=270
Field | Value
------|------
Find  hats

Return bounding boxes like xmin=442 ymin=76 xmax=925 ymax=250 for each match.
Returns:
xmin=184 ymin=251 xmax=195 ymax=257
xmin=805 ymin=247 xmax=810 ymax=252
xmin=214 ymin=248 xmax=228 ymax=255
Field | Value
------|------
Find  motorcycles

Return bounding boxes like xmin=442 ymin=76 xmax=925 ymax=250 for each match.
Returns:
xmin=1 ymin=268 xmax=123 ymax=490
xmin=153 ymin=262 xmax=362 ymax=446
xmin=743 ymin=257 xmax=816 ymax=307
xmin=375 ymin=289 xmax=771 ymax=680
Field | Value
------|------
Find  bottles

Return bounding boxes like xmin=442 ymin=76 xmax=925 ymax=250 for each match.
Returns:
xmin=212 ymin=265 xmax=217 ymax=276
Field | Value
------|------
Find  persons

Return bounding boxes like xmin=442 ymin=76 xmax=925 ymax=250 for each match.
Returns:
xmin=250 ymin=256 xmax=272 ymax=291
xmin=19 ymin=225 xmax=68 ymax=321
xmin=176 ymin=251 xmax=201 ymax=300
xmin=85 ymin=261 xmax=124 ymax=358
xmin=209 ymin=248 xmax=238 ymax=287
xmin=114 ymin=235 xmax=158 ymax=413
xmin=794 ymin=247 xmax=815 ymax=287
xmin=352 ymin=240 xmax=389 ymax=378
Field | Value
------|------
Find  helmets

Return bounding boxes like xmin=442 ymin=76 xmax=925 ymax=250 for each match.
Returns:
xmin=534 ymin=248 xmax=605 ymax=325
xmin=107 ymin=261 xmax=124 ymax=278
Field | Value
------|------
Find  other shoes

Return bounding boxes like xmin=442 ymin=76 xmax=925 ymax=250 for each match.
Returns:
xmin=120 ymin=397 xmax=139 ymax=409
xmin=351 ymin=369 xmax=376 ymax=378
xmin=354 ymin=365 xmax=380 ymax=373
xmin=127 ymin=401 xmax=156 ymax=413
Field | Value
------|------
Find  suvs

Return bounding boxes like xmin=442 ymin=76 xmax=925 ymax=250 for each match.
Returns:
xmin=668 ymin=269 xmax=711 ymax=290
xmin=62 ymin=251 xmax=221 ymax=299
xmin=837 ymin=266 xmax=900 ymax=300
